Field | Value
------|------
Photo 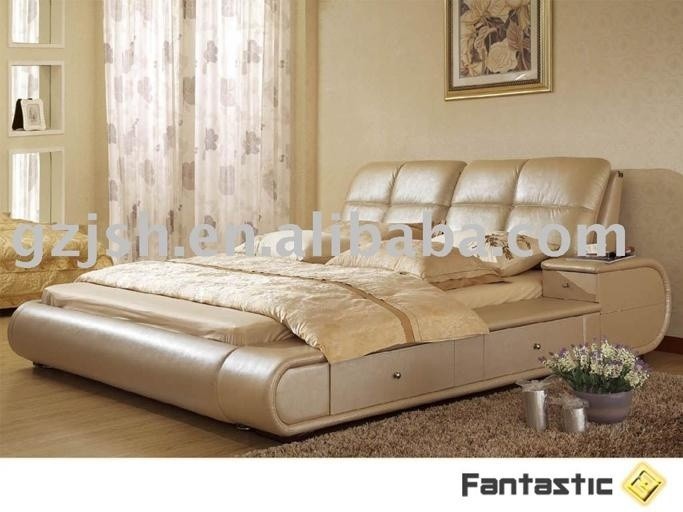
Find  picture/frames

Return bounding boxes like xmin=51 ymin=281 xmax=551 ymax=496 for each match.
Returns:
xmin=18 ymin=99 xmax=46 ymax=131
xmin=442 ymin=0 xmax=556 ymax=102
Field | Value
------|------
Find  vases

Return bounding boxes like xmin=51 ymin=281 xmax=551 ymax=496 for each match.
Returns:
xmin=575 ymin=390 xmax=631 ymax=424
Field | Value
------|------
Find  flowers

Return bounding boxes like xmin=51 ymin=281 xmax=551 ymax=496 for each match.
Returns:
xmin=536 ymin=337 xmax=653 ymax=394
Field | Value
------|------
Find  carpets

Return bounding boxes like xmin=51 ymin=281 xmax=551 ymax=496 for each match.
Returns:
xmin=237 ymin=371 xmax=682 ymax=456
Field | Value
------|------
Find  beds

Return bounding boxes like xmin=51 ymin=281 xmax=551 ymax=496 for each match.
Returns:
xmin=6 ymin=160 xmax=669 ymax=439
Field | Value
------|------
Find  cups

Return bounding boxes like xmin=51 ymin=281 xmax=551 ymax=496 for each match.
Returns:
xmin=524 ymin=388 xmax=548 ymax=431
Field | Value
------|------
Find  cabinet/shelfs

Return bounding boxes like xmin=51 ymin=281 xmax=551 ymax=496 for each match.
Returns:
xmin=0 ymin=1 xmax=106 ymax=239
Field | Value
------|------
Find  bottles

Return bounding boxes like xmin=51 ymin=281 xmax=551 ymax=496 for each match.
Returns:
xmin=561 ymin=406 xmax=587 ymax=432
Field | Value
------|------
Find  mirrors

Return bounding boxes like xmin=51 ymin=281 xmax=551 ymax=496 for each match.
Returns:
xmin=7 ymin=152 xmax=50 ymax=224
xmin=6 ymin=0 xmax=50 ymax=44
xmin=10 ymin=65 xmax=50 ymax=131
